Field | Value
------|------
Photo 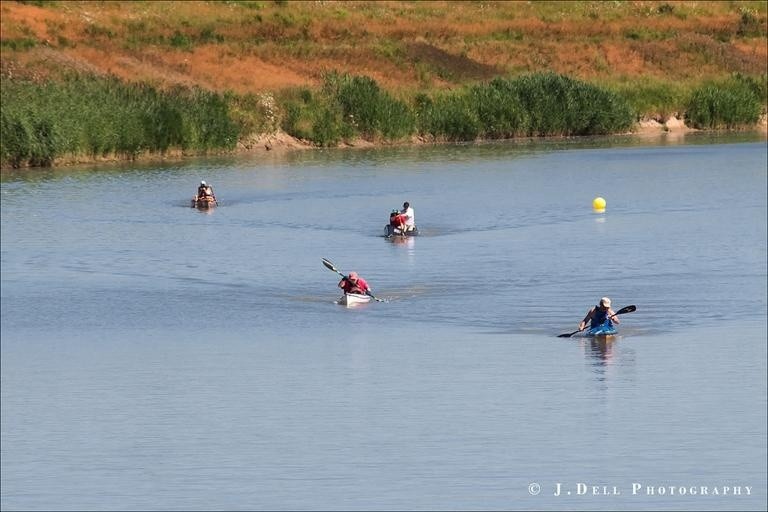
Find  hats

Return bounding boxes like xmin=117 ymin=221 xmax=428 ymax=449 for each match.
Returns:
xmin=599 ymin=297 xmax=611 ymax=308
xmin=391 ymin=207 xmax=401 ymax=214
xmin=349 ymin=271 xmax=358 ymax=280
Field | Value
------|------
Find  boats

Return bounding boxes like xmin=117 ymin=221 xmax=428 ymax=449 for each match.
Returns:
xmin=383 ymin=224 xmax=419 ymax=238
xmin=190 ymin=197 xmax=216 ymax=211
xmin=337 ymin=292 xmax=371 ymax=307
xmin=582 ymin=321 xmax=620 ymax=336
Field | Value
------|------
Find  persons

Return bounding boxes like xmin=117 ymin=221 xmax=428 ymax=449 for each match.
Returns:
xmin=337 ymin=271 xmax=372 ymax=296
xmin=578 ymin=297 xmax=621 ymax=331
xmin=197 ymin=181 xmax=212 ymax=199
xmin=393 ymin=200 xmax=414 ymax=232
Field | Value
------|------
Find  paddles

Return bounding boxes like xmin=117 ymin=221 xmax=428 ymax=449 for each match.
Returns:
xmin=322 ymin=258 xmax=383 ymax=302
xmin=557 ymin=305 xmax=636 ymax=337
